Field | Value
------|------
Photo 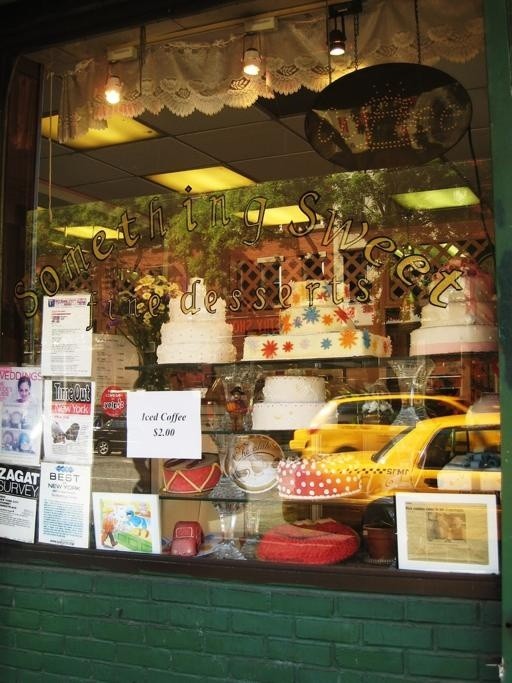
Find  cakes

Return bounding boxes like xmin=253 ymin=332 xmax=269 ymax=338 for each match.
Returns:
xmin=250 ymin=375 xmax=339 ymax=431
xmin=242 ymin=279 xmax=393 ymax=360
xmin=162 ymin=451 xmax=221 ymax=494
xmin=156 ymin=277 xmax=238 ymax=364
xmin=275 ymin=454 xmax=362 ymax=502
xmin=408 ymin=256 xmax=499 ymax=355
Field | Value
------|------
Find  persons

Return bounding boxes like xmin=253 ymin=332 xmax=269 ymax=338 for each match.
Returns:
xmin=15 ymin=376 xmax=31 ymax=403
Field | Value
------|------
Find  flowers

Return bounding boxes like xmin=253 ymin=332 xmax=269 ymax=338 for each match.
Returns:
xmin=105 ymin=269 xmax=181 ymax=354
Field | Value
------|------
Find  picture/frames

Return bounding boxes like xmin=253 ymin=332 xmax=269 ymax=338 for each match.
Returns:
xmin=393 ymin=492 xmax=499 ymax=575
xmin=92 ymin=491 xmax=161 ymax=554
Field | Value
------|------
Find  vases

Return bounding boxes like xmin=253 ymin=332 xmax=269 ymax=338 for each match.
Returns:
xmin=362 ymin=521 xmax=398 ymax=565
xmin=133 ymin=351 xmax=170 ymax=391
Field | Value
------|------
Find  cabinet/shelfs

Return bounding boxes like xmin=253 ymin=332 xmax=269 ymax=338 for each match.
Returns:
xmin=110 ymin=349 xmax=502 ymax=505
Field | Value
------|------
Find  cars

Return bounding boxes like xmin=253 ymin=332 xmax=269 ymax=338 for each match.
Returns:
xmin=93 ymin=416 xmax=127 ymax=456
xmin=214 ymin=364 xmax=265 ymax=395
xmin=282 ymin=391 xmax=502 ymax=545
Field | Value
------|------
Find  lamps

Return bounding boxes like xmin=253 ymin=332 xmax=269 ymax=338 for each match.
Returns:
xmin=238 ymin=17 xmax=275 ymax=74
xmin=326 ymin=0 xmax=363 ymax=55
xmin=103 ymin=46 xmax=138 ymax=104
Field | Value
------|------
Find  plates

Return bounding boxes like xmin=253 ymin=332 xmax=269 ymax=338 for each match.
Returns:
xmin=224 ymin=434 xmax=286 ymax=493
